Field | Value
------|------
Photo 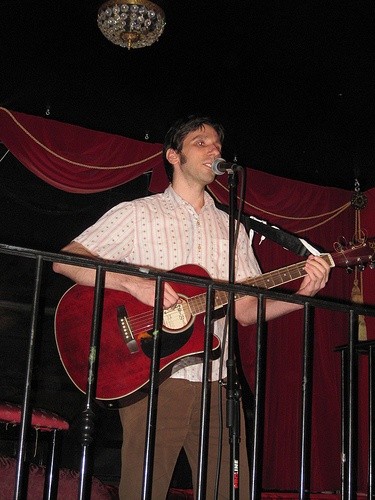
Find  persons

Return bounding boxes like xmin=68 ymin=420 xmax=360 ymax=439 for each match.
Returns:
xmin=53 ymin=114 xmax=331 ymax=500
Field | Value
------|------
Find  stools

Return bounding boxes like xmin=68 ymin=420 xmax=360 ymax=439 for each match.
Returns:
xmin=0 ymin=401 xmax=70 ymax=500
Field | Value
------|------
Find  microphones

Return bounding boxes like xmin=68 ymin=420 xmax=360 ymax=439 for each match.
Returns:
xmin=212 ymin=158 xmax=245 ymax=176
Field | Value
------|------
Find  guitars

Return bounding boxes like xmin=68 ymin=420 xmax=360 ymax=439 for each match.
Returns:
xmin=54 ymin=233 xmax=375 ymax=408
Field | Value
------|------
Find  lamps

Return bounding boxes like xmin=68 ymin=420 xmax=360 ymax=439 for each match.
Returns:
xmin=96 ymin=0 xmax=166 ymax=51
